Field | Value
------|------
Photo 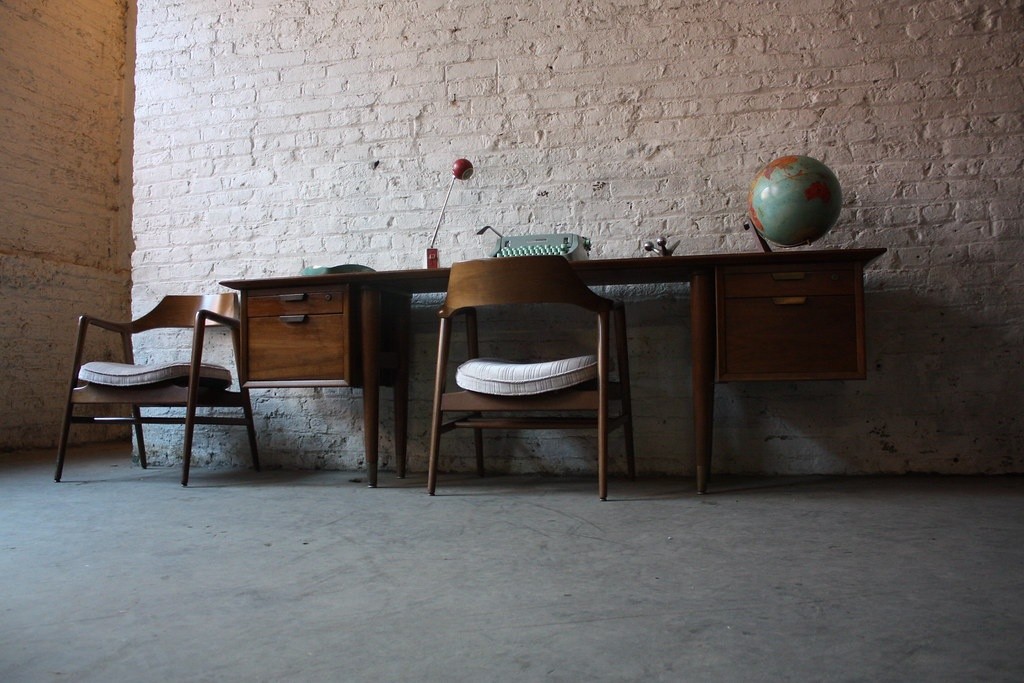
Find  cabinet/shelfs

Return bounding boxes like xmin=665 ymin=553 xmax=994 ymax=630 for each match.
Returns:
xmin=711 ymin=262 xmax=868 ymax=383
xmin=239 ymin=284 xmax=403 ymax=388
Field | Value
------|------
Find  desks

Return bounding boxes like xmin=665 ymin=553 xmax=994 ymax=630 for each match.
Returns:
xmin=218 ymin=246 xmax=888 ymax=495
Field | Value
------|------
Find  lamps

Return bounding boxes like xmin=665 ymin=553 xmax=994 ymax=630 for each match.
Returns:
xmin=426 ymin=158 xmax=473 ymax=268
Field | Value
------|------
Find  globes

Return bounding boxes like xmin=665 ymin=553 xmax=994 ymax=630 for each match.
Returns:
xmin=747 ymin=154 xmax=843 ymax=252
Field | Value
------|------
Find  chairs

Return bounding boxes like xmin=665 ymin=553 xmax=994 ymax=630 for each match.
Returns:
xmin=426 ymin=255 xmax=636 ymax=501
xmin=54 ymin=292 xmax=259 ymax=487
